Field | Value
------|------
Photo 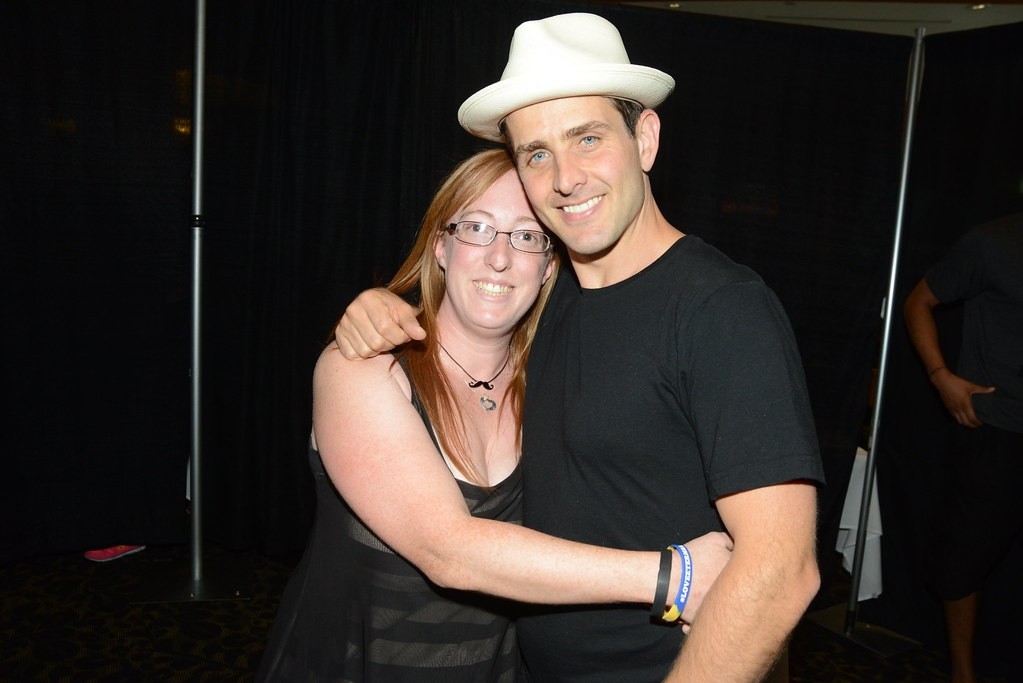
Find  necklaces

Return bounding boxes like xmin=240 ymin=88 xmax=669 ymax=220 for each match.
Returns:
xmin=440 ymin=353 xmax=496 ymax=412
xmin=435 ymin=338 xmax=511 ymax=390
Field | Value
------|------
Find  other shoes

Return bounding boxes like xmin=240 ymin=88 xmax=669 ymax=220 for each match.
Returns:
xmin=84 ymin=545 xmax=146 ymax=562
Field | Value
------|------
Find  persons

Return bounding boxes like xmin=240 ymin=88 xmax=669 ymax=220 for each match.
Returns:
xmin=336 ymin=10 xmax=826 ymax=683
xmin=904 ymin=213 xmax=1023 ymax=683
xmin=249 ymin=148 xmax=733 ymax=683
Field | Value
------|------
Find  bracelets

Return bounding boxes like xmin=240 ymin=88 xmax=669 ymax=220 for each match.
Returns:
xmin=662 ymin=545 xmax=691 ymax=622
xmin=930 ymin=366 xmax=946 ymax=375
xmin=650 ymin=549 xmax=672 ymax=621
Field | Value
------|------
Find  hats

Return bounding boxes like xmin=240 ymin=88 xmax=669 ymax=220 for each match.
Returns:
xmin=457 ymin=13 xmax=675 ymax=143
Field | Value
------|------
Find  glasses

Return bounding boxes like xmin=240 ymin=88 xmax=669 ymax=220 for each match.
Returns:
xmin=442 ymin=221 xmax=554 ymax=254
